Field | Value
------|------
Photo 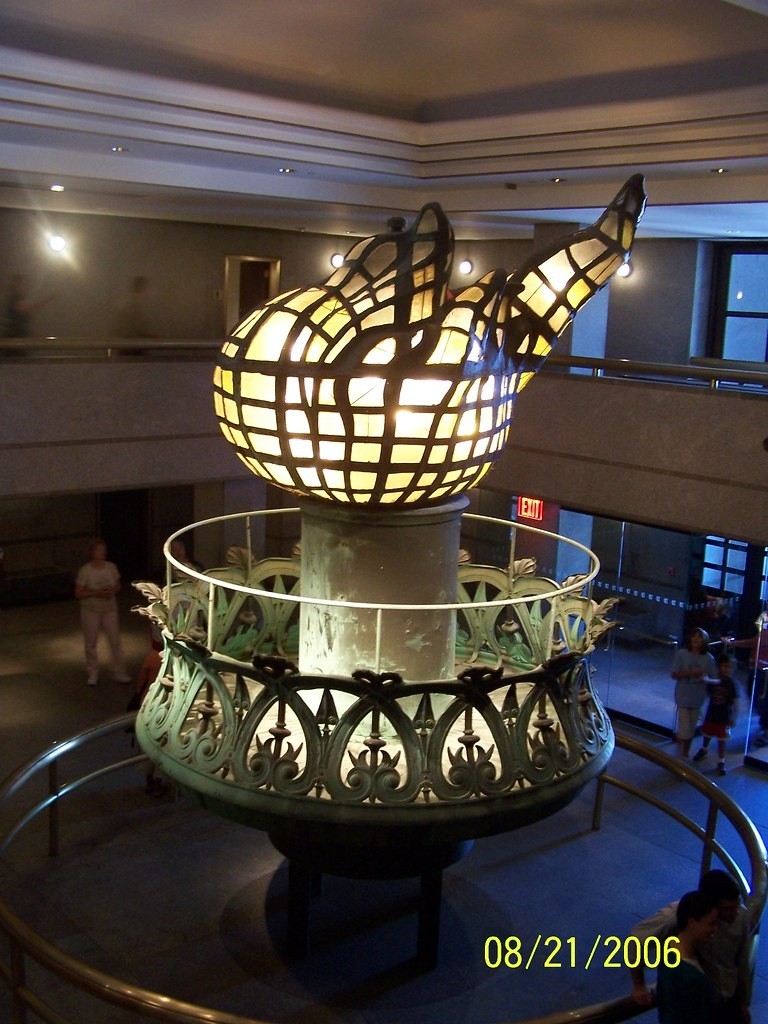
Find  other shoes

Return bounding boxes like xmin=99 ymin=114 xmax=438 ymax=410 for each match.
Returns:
xmin=691 ymin=748 xmax=708 ymax=761
xmin=717 ymin=759 xmax=727 ymax=775
xmin=88 ymin=673 xmax=98 ymax=685
xmin=111 ymin=673 xmax=131 ymax=682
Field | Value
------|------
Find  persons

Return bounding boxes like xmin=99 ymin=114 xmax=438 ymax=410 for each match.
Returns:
xmin=74 ymin=536 xmax=200 ymax=740
xmin=667 ymin=628 xmax=740 ymax=774
xmin=624 ymin=868 xmax=768 ymax=1024
